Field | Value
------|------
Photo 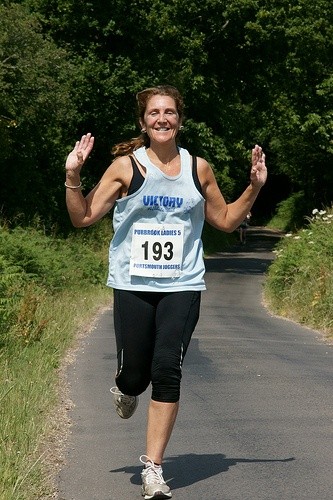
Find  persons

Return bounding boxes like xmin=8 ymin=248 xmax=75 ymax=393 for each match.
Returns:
xmin=62 ymin=84 xmax=268 ymax=500
xmin=238 ymin=215 xmax=248 ymax=246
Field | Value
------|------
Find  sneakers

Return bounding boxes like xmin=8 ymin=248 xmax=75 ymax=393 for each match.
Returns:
xmin=139 ymin=454 xmax=172 ymax=499
xmin=109 ymin=385 xmax=137 ymax=420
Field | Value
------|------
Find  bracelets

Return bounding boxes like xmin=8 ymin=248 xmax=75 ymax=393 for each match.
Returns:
xmin=64 ymin=181 xmax=82 ymax=189
